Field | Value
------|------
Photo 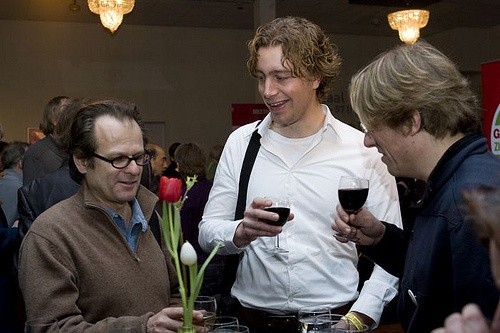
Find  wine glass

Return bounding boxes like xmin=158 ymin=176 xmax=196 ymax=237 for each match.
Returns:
xmin=333 ymin=175 xmax=369 ymax=242
xmin=258 ymin=200 xmax=290 ymax=254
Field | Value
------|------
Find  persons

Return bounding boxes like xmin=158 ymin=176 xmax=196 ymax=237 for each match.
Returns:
xmin=331 ymin=38 xmax=500 ymax=333
xmin=198 ymin=16 xmax=404 ymax=333
xmin=0 ymin=95 xmax=225 ymax=333
xmin=17 ymin=99 xmax=209 ymax=333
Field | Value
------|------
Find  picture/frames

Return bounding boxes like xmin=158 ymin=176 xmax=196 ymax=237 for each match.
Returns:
xmin=27 ymin=127 xmax=46 ymax=145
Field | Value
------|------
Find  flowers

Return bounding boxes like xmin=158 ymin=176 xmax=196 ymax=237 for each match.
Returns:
xmin=155 ymin=174 xmax=221 ymax=333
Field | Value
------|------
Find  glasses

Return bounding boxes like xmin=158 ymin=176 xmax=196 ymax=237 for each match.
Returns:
xmin=359 ymin=109 xmax=428 ymax=134
xmin=93 ymin=152 xmax=151 ymax=169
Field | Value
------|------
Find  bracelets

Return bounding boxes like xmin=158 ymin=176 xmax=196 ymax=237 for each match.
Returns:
xmin=345 ymin=313 xmax=363 ymax=330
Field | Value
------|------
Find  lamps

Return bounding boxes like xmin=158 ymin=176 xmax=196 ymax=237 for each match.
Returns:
xmin=387 ymin=3 xmax=430 ymax=47
xmin=86 ymin=0 xmax=135 ymax=33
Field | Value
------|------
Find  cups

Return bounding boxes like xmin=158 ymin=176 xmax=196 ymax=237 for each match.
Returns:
xmin=180 ymin=296 xmax=249 ymax=333
xmin=299 ymin=304 xmax=369 ymax=333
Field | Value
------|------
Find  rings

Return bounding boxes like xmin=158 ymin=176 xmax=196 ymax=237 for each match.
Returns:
xmin=333 ymin=234 xmax=338 ymax=238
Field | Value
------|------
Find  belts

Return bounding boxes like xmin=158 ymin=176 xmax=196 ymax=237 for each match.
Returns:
xmin=238 ymin=305 xmax=352 ymax=333
